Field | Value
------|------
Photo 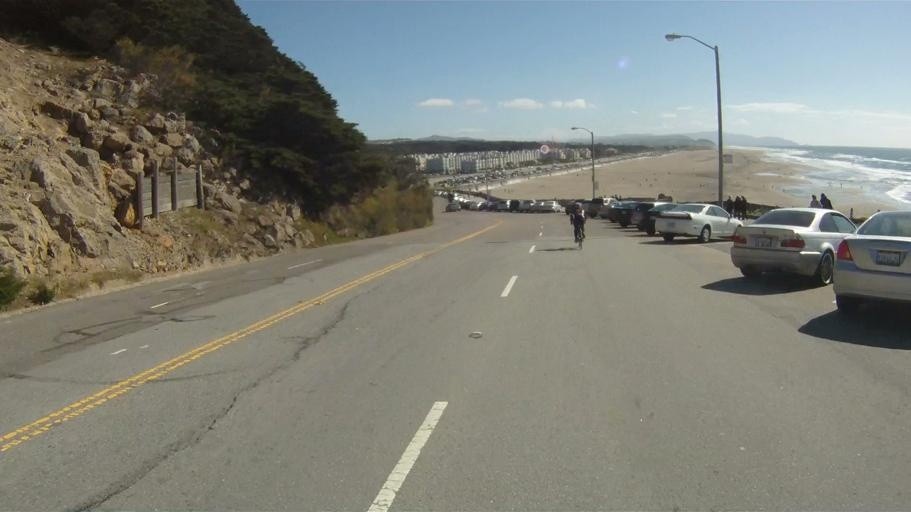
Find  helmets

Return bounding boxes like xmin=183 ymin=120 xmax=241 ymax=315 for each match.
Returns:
xmin=574 ymin=202 xmax=581 ymax=207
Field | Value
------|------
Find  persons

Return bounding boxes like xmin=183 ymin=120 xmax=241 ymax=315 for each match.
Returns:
xmin=733 ymin=196 xmax=741 ymax=220
xmin=611 ymin=193 xmax=621 ymax=201
xmin=820 ymin=193 xmax=833 ymax=209
xmin=726 ymin=195 xmax=733 ymax=214
xmin=810 ymin=195 xmax=823 ymax=208
xmin=741 ymin=195 xmax=748 ymax=221
xmin=569 ymin=201 xmax=586 ymax=243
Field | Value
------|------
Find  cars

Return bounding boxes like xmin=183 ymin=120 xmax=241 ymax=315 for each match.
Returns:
xmin=730 ymin=208 xmax=860 ymax=285
xmin=432 ymin=190 xmax=671 ymax=237
xmin=833 ymin=210 xmax=911 ymax=313
xmin=433 ymin=162 xmax=576 ymax=189
xmin=655 ymin=203 xmax=744 ymax=244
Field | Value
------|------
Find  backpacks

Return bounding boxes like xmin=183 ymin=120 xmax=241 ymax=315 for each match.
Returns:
xmin=575 ymin=208 xmax=584 ymax=219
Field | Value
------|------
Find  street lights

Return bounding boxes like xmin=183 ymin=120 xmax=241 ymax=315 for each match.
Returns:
xmin=664 ymin=33 xmax=724 ymax=207
xmin=571 ymin=127 xmax=595 ymax=199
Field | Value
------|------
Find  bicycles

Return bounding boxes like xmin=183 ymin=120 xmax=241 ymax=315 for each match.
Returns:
xmin=576 ymin=222 xmax=585 ymax=249
